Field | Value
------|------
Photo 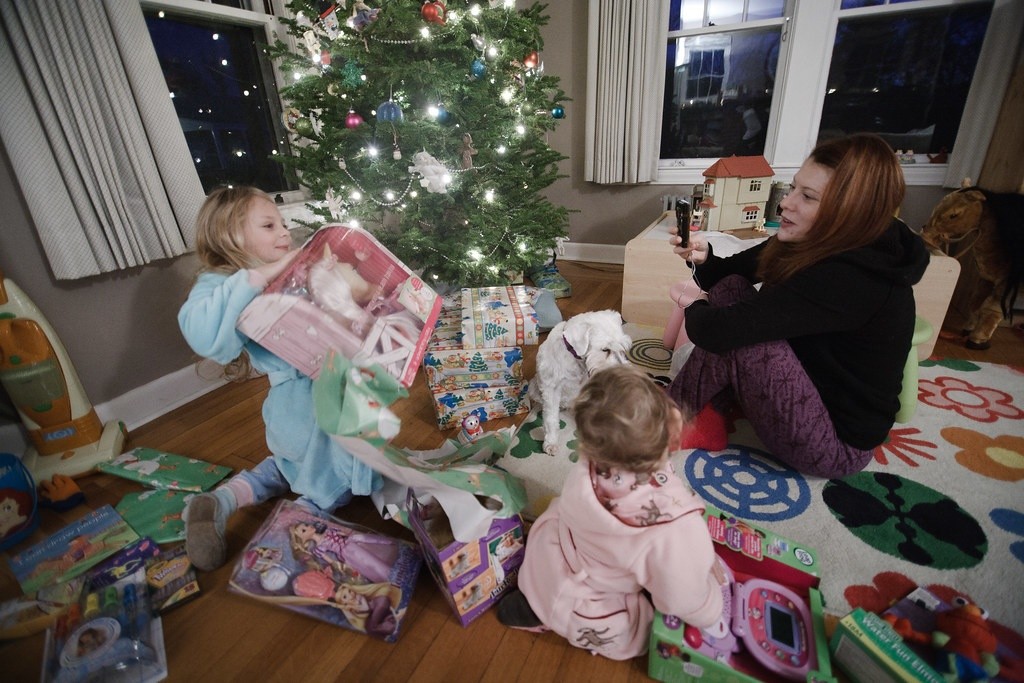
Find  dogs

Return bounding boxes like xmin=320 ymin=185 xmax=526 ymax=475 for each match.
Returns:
xmin=526 ymin=309 xmax=633 ymax=457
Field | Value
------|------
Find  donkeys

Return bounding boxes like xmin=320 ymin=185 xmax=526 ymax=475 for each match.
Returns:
xmin=921 ymin=176 xmax=1024 ymax=350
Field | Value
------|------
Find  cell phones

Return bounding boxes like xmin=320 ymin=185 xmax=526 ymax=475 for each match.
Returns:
xmin=676 ymin=200 xmax=690 ymax=247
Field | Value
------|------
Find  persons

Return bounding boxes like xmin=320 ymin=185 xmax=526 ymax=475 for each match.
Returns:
xmin=671 ymin=134 xmax=928 ymax=476
xmin=496 ymin=369 xmax=730 ymax=660
xmin=77 ymin=628 xmax=106 ymax=656
xmin=287 ymin=524 xmax=396 ymax=584
xmin=176 ymin=184 xmax=383 ymax=573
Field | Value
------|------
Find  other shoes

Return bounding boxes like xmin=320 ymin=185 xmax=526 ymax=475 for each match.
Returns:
xmin=186 ymin=493 xmax=227 ymax=571
xmin=495 ymin=589 xmax=552 ymax=634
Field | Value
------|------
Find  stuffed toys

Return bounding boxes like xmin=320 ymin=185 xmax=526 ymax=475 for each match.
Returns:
xmin=882 ymin=596 xmax=1002 ymax=683
xmin=919 ymin=177 xmax=1023 ymax=350
